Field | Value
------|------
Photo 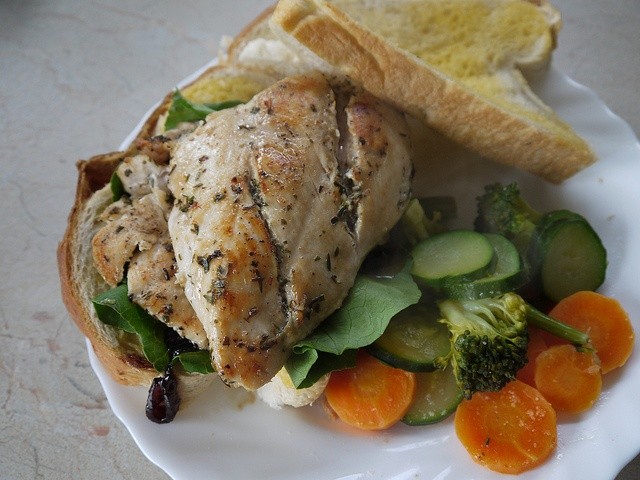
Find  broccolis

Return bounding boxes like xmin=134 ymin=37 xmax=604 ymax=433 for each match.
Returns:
xmin=400 ymin=197 xmax=446 ymax=253
xmin=434 ymin=292 xmax=530 ymax=404
xmin=474 ymin=181 xmax=539 ymax=242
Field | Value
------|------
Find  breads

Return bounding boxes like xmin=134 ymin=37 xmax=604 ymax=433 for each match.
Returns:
xmin=56 ymin=0 xmax=334 ymax=409
xmin=267 ymin=0 xmax=598 ymax=185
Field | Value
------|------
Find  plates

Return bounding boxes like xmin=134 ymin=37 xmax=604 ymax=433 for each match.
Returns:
xmin=85 ymin=54 xmax=640 ymax=479
